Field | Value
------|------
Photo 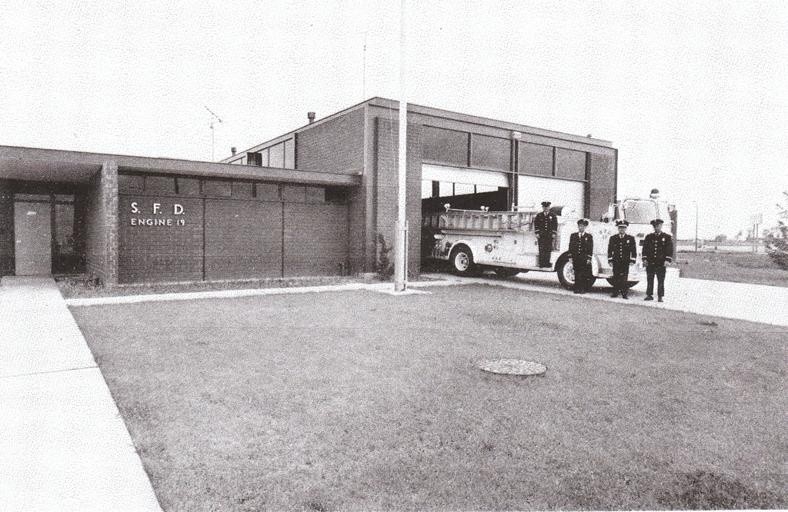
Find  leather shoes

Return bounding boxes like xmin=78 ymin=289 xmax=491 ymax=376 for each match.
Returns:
xmin=644 ymin=296 xmax=663 ymax=302
xmin=574 ymin=289 xmax=585 ymax=293
xmin=541 ymin=264 xmax=552 ymax=267
xmin=611 ymin=295 xmax=628 ymax=299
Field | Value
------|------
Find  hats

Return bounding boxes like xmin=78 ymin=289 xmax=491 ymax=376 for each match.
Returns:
xmin=578 ymin=219 xmax=588 ymax=226
xmin=616 ymin=220 xmax=629 ymax=226
xmin=651 ymin=220 xmax=663 ymax=225
xmin=542 ymin=202 xmax=551 ymax=206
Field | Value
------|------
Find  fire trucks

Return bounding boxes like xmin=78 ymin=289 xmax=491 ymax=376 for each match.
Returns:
xmin=421 ymin=183 xmax=681 ymax=292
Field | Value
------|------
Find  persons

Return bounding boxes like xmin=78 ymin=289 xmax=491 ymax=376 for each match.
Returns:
xmin=642 ymin=219 xmax=673 ymax=302
xmin=606 ymin=219 xmax=637 ymax=299
xmin=567 ymin=219 xmax=593 ymax=294
xmin=535 ymin=200 xmax=558 ymax=268
